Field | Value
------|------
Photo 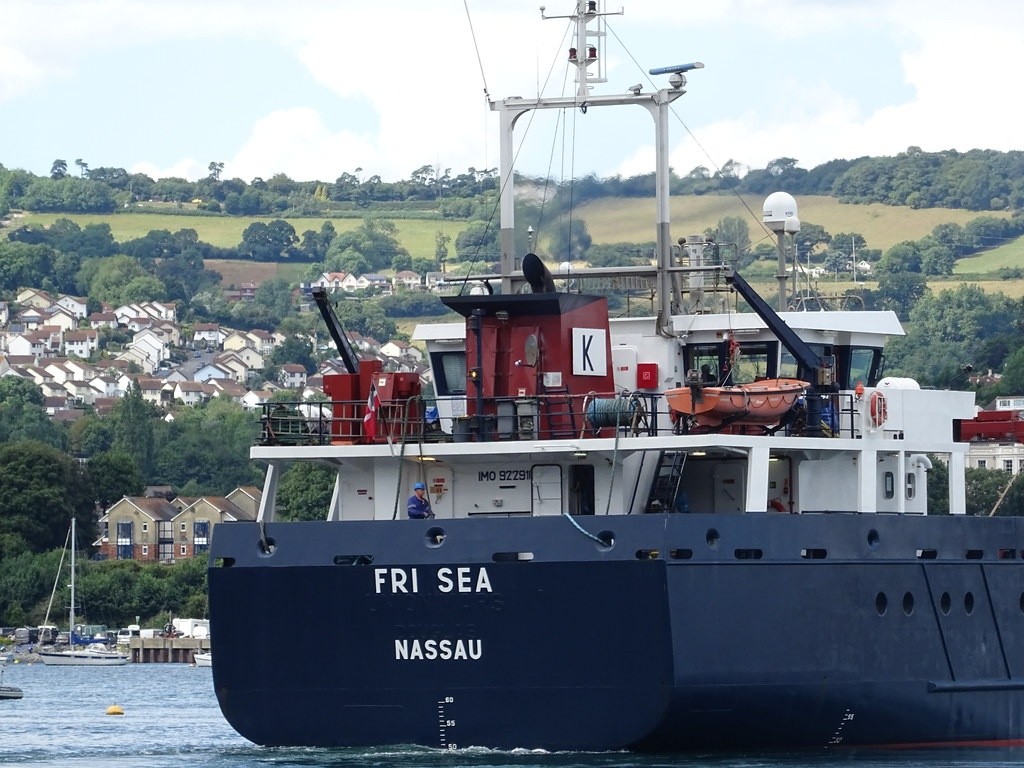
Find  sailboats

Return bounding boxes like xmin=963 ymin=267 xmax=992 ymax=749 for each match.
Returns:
xmin=34 ymin=516 xmax=129 ymax=666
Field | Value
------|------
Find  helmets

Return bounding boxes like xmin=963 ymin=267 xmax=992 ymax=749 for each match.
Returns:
xmin=414 ymin=482 xmax=426 ymax=490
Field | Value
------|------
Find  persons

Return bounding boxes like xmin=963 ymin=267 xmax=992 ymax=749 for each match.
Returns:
xmin=700 ymin=362 xmax=717 ymax=384
xmin=405 ymin=483 xmax=434 ymax=522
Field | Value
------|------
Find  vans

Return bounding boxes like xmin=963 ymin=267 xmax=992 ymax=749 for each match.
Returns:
xmin=117 ymin=629 xmax=142 ymax=645
xmin=0 ymin=625 xmax=60 ymax=644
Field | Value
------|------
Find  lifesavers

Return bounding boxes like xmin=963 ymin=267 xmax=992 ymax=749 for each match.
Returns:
xmin=869 ymin=391 xmax=888 ymax=426
xmin=767 ymin=499 xmax=785 ymax=514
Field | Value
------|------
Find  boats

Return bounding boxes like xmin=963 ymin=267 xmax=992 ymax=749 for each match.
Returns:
xmin=201 ymin=1 xmax=1024 ymax=757
xmin=193 ymin=649 xmax=212 ymax=667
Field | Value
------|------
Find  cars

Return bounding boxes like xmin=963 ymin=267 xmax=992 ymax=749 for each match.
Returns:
xmin=55 ymin=634 xmax=70 ymax=644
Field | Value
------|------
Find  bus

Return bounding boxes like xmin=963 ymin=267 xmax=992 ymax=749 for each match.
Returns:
xmin=73 ymin=623 xmax=108 ymax=644
